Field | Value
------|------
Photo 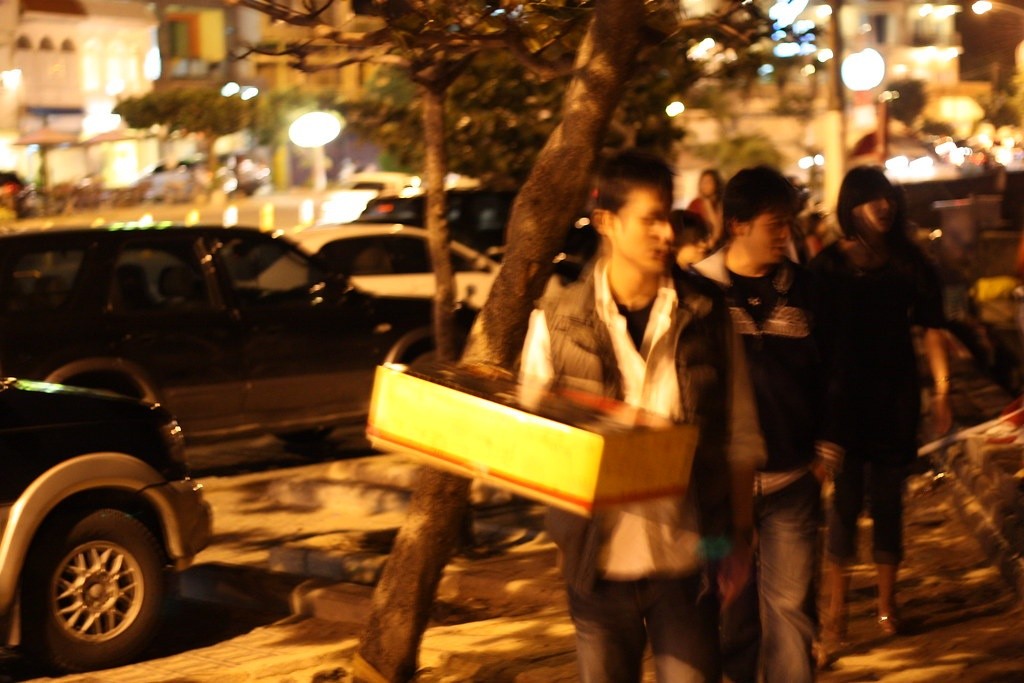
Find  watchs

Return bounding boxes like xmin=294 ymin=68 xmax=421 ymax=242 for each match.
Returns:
xmin=731 ymin=526 xmax=760 ymax=546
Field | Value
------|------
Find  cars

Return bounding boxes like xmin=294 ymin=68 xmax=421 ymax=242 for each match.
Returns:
xmin=1 ymin=225 xmax=472 ymax=447
xmin=289 ymin=221 xmax=498 ymax=308
xmin=350 ymin=171 xmax=515 ymax=250
xmin=0 ymin=374 xmax=216 ymax=673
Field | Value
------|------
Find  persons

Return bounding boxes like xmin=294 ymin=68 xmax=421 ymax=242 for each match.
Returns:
xmin=521 ymin=162 xmax=852 ymax=316
xmin=684 ymin=166 xmax=857 ymax=682
xmin=512 ymin=151 xmax=770 ymax=683
xmin=799 ymin=164 xmax=954 ymax=644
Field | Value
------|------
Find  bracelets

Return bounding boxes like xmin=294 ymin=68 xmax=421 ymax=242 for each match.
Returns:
xmin=931 ymin=375 xmax=953 ymax=385
xmin=934 ymin=390 xmax=950 ymax=397
